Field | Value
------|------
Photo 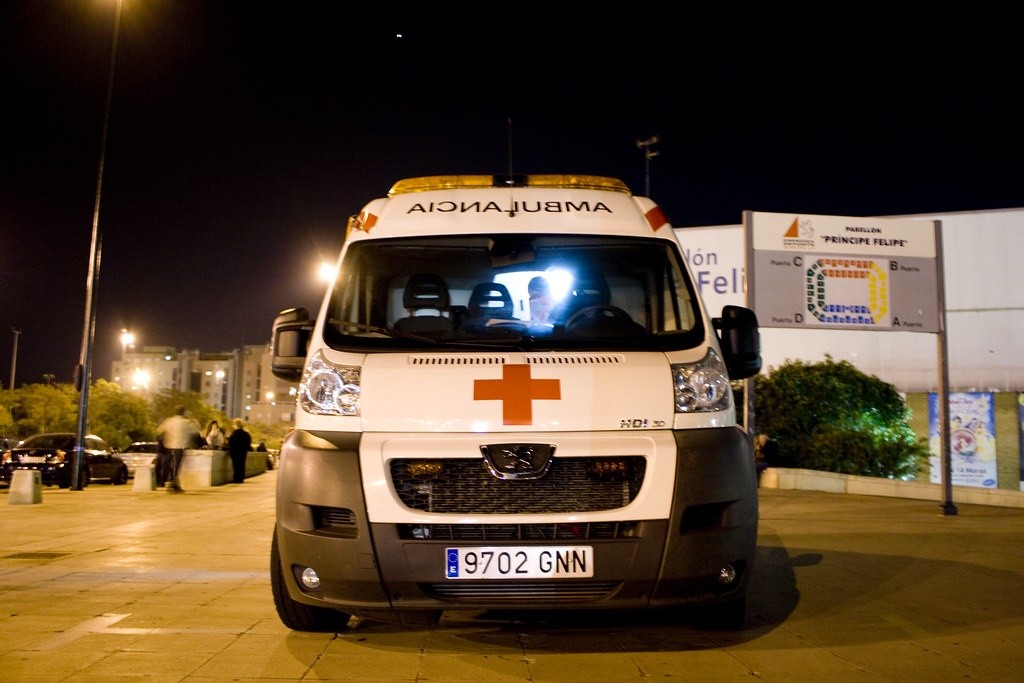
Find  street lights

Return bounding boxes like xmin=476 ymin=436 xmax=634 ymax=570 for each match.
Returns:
xmin=8 ymin=325 xmax=23 ymax=390
xmin=119 ymin=328 xmax=134 ymax=390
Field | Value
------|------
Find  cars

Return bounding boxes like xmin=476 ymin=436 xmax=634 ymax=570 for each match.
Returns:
xmin=0 ymin=437 xmax=22 ymax=468
xmin=111 ymin=441 xmax=158 ymax=478
xmin=2 ymin=431 xmax=128 ymax=490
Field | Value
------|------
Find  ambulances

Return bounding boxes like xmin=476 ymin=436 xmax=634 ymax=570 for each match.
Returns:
xmin=268 ymin=171 xmax=765 ymax=634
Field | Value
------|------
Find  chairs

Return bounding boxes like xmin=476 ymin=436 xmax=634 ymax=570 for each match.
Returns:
xmin=460 ymin=282 xmax=521 ymax=324
xmin=392 ymin=272 xmax=453 ymax=334
xmin=555 ymin=277 xmax=613 ymax=321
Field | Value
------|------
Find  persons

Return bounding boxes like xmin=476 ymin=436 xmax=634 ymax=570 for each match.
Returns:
xmin=228 ymin=418 xmax=251 ymax=484
xmin=188 ymin=418 xmax=204 ymax=450
xmin=204 ymin=421 xmax=225 ymax=450
xmin=157 ymin=404 xmax=200 ymax=491
xmin=754 ymin=434 xmax=770 ymax=486
xmin=256 ymin=441 xmax=267 ymax=452
